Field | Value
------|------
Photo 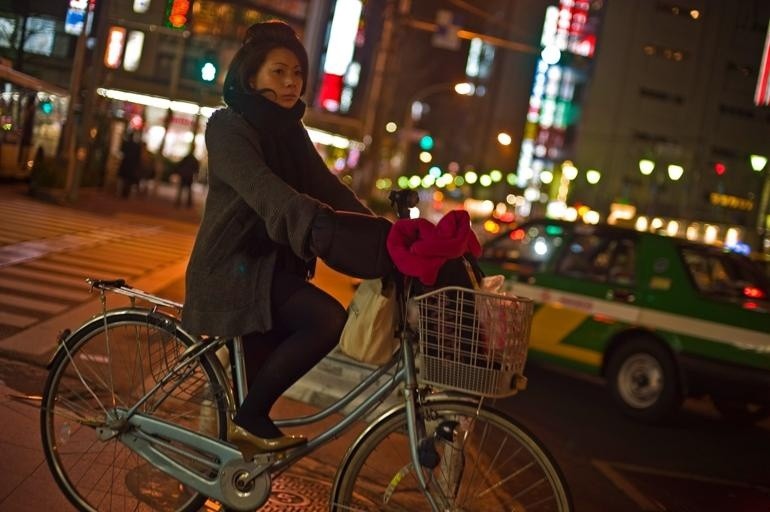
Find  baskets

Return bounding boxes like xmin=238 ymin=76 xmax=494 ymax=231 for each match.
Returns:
xmin=414 ymin=285 xmax=536 ymax=401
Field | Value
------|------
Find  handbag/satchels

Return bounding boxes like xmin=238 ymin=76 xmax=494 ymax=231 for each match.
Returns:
xmin=338 ymin=276 xmax=401 ymax=366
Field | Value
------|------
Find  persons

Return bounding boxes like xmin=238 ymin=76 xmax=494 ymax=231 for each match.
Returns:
xmin=177 ymin=19 xmax=405 ymax=457
xmin=172 ymin=142 xmax=200 ymax=210
xmin=139 ymin=140 xmax=158 ymax=181
xmin=116 ymin=131 xmax=143 ymax=199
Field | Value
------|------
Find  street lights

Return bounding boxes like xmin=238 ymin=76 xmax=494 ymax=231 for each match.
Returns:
xmin=749 ymin=154 xmax=769 ymax=247
xmin=639 ymin=153 xmax=684 ymax=231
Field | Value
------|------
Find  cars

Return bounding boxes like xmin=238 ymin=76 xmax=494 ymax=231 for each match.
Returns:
xmin=478 ymin=218 xmax=769 ymax=423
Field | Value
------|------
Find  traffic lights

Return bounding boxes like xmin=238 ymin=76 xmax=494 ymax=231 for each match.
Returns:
xmin=195 ymin=46 xmax=221 ymax=87
xmin=534 ymin=43 xmax=594 ymax=76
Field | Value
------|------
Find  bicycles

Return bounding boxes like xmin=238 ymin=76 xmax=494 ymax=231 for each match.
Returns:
xmin=40 ymin=190 xmax=576 ymax=512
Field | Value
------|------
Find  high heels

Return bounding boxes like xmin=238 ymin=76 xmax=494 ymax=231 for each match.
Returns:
xmin=226 ymin=415 xmax=308 ymax=463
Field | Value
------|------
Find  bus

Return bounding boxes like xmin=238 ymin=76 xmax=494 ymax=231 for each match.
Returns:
xmin=0 ymin=58 xmax=69 ymax=193
